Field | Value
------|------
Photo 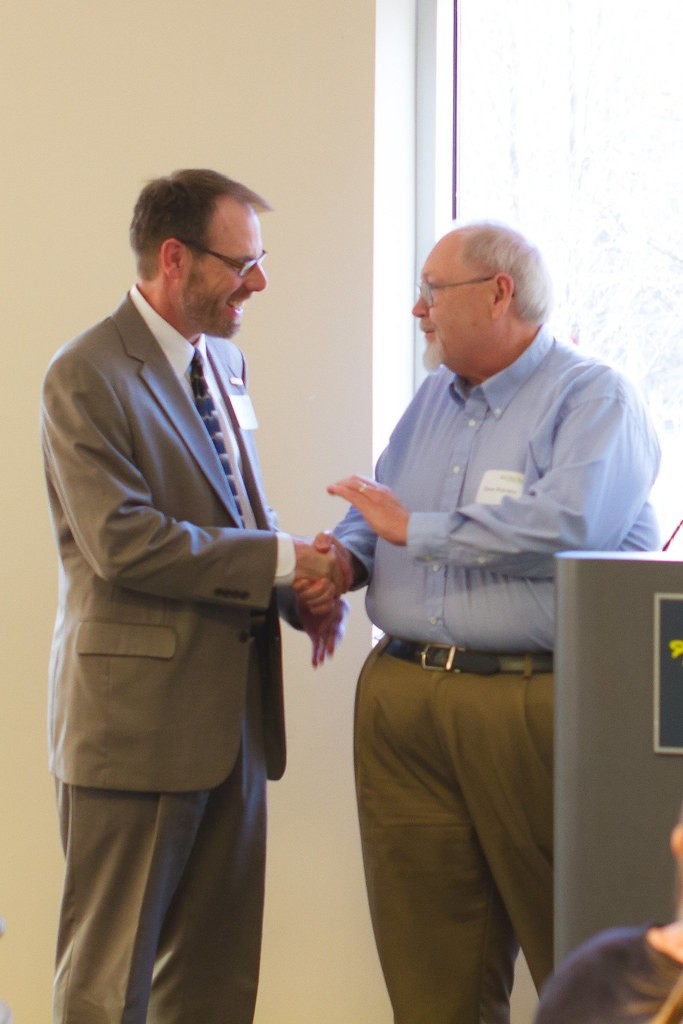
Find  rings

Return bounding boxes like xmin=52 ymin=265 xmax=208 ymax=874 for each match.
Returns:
xmin=358 ymin=484 xmax=368 ymax=493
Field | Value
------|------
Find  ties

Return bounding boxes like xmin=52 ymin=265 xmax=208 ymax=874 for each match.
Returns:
xmin=189 ymin=347 xmax=247 ymax=530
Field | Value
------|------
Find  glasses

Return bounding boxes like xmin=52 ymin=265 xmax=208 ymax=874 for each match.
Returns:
xmin=414 ymin=276 xmax=497 ymax=308
xmin=174 ymin=236 xmax=267 ymax=278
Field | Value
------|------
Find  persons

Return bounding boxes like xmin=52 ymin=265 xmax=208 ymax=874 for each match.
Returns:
xmin=534 ymin=802 xmax=683 ymax=1024
xmin=37 ymin=165 xmax=350 ymax=1024
xmin=294 ymin=216 xmax=664 ymax=1023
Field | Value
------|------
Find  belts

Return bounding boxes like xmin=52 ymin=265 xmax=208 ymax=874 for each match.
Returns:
xmin=383 ymin=636 xmax=555 ymax=676
